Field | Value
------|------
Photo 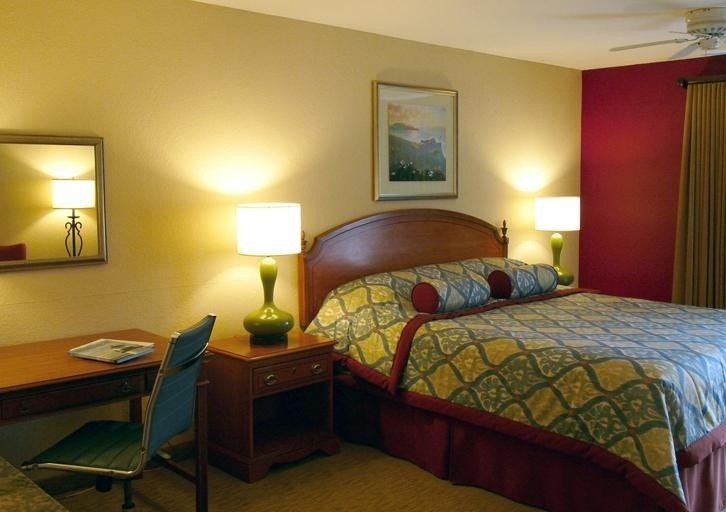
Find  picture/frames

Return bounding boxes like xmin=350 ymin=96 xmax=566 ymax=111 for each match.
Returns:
xmin=372 ymin=81 xmax=458 ymax=201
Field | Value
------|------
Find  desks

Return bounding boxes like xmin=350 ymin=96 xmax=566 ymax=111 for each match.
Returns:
xmin=0 ymin=328 xmax=217 ymax=512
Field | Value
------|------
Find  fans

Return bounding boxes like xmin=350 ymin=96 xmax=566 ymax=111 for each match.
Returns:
xmin=610 ymin=8 xmax=726 ymax=60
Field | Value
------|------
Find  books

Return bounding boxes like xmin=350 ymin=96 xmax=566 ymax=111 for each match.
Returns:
xmin=67 ymin=338 xmax=156 ymax=364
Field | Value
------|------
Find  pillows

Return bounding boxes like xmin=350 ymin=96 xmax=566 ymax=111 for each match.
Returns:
xmin=411 ymin=273 xmax=490 ymax=312
xmin=488 ymin=264 xmax=558 ymax=300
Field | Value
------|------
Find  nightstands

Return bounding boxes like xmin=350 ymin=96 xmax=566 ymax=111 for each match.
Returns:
xmin=205 ymin=331 xmax=338 ymax=483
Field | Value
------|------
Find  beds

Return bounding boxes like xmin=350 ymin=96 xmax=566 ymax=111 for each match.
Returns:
xmin=298 ymin=208 xmax=726 ymax=511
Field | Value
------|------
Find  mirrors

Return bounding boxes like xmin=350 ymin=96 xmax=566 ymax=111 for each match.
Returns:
xmin=0 ymin=128 xmax=107 ymax=273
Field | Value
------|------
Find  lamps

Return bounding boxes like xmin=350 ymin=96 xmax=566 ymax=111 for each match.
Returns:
xmin=236 ymin=203 xmax=302 ymax=345
xmin=535 ymin=195 xmax=581 ymax=286
xmin=52 ymin=179 xmax=96 ymax=257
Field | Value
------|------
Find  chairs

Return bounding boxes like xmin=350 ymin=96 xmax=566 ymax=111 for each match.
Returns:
xmin=0 ymin=244 xmax=27 ymax=261
xmin=21 ymin=314 xmax=216 ymax=510
